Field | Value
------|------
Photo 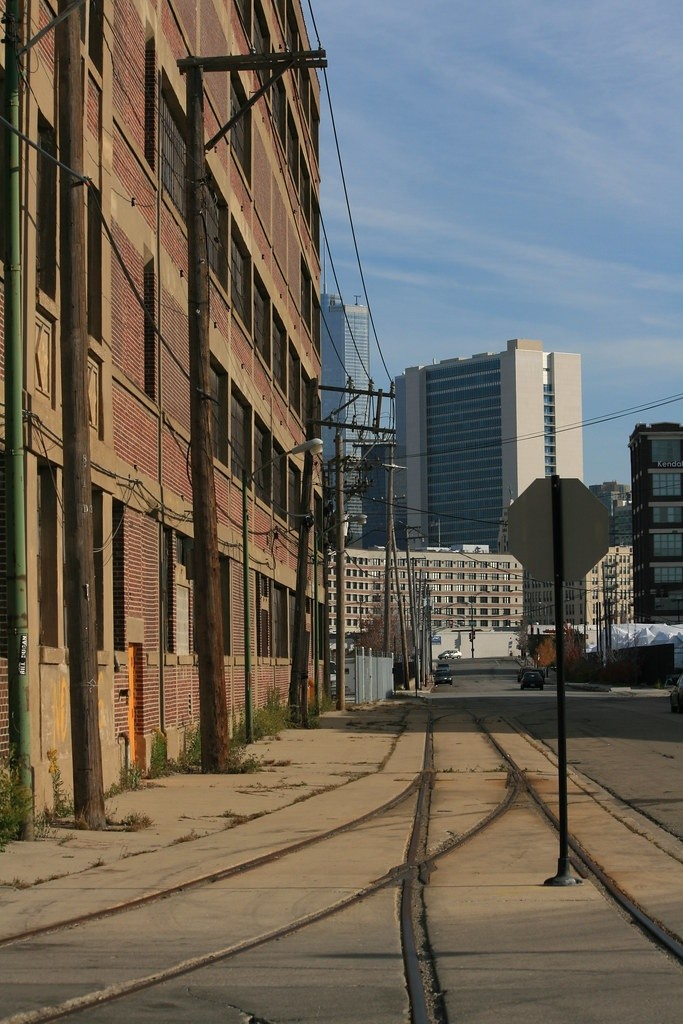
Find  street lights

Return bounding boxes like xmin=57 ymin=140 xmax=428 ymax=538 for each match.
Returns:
xmin=313 ymin=513 xmax=368 ymax=715
xmin=241 ymin=437 xmax=325 ymax=744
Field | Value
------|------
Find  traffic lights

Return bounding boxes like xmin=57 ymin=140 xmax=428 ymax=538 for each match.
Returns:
xmin=473 ymin=631 xmax=475 ymax=640
xmin=468 ymin=632 xmax=471 ymax=639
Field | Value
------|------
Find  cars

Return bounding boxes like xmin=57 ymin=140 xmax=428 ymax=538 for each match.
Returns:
xmin=669 ymin=674 xmax=683 ymax=713
xmin=438 ymin=650 xmax=463 ymax=659
xmin=516 ymin=666 xmax=546 ymax=690
xmin=433 ymin=671 xmax=453 ymax=685
xmin=435 ymin=663 xmax=451 ymax=672
